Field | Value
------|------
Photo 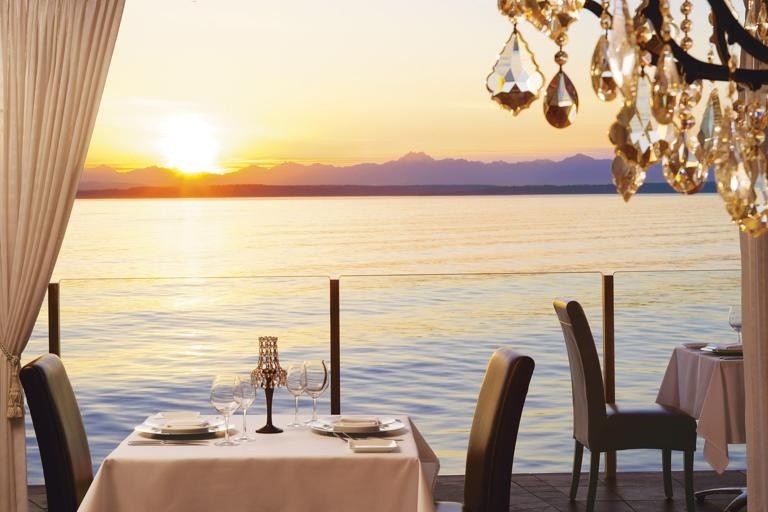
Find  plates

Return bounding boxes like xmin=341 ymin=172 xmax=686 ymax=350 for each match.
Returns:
xmin=347 ymin=439 xmax=398 ymax=452
xmin=682 ymin=342 xmax=742 ymax=354
xmin=134 ymin=411 xmax=233 ymax=435
xmin=309 ymin=414 xmax=405 ymax=435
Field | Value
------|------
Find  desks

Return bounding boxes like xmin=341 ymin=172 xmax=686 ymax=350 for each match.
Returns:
xmin=655 ymin=342 xmax=747 ymax=511
xmin=73 ymin=412 xmax=441 ymax=511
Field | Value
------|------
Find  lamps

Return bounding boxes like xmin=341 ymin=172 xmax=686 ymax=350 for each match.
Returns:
xmin=477 ymin=1 xmax=763 ymax=239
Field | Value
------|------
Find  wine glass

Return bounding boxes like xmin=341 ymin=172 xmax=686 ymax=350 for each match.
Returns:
xmin=286 ymin=358 xmax=329 ymax=429
xmin=210 ymin=373 xmax=256 ymax=447
xmin=727 ymin=304 xmax=742 ymax=344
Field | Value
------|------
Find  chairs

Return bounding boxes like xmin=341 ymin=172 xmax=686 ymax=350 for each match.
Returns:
xmin=552 ymin=298 xmax=697 ymax=510
xmin=21 ymin=353 xmax=94 ymax=510
xmin=434 ymin=346 xmax=536 ymax=512
